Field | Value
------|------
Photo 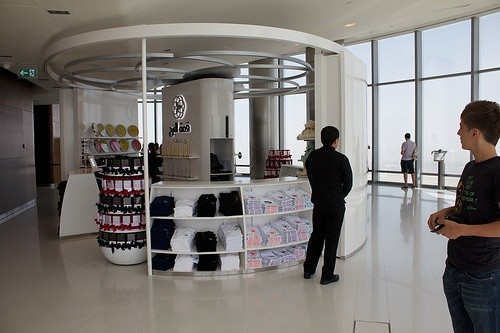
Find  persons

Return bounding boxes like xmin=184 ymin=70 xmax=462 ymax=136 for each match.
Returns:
xmin=401 ymin=133 xmax=416 ymax=189
xmin=428 ymin=100 xmax=500 ymax=333
xmin=303 ymin=125 xmax=353 ymax=284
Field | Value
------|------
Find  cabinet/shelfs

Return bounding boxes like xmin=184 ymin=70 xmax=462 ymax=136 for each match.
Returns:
xmin=41 ymin=23 xmax=367 ymax=276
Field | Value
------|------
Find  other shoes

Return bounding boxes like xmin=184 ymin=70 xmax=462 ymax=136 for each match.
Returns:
xmin=320 ymin=274 xmax=339 ymax=285
xmin=304 ymin=273 xmax=311 ymax=279
